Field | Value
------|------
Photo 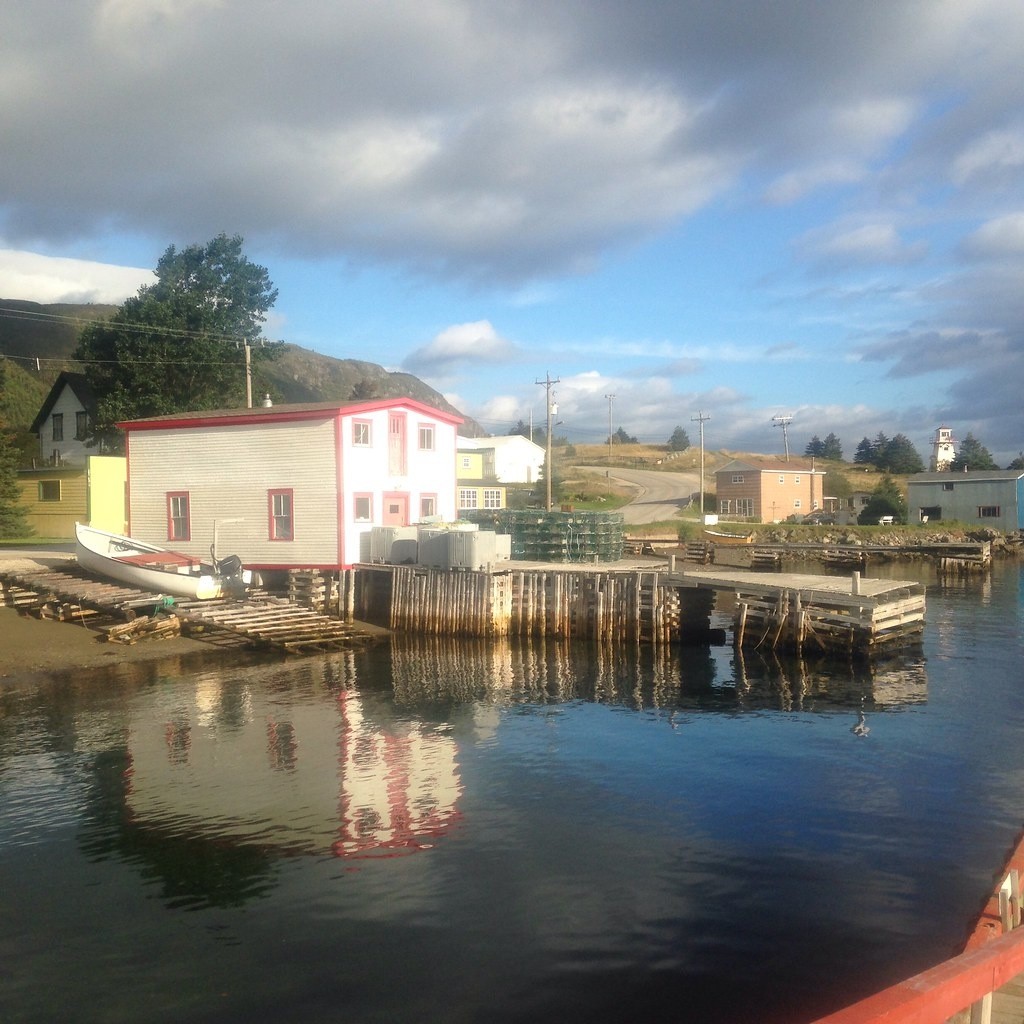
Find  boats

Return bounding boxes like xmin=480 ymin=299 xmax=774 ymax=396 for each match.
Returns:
xmin=701 ymin=529 xmax=753 ymax=544
xmin=74 ymin=519 xmax=263 ymax=600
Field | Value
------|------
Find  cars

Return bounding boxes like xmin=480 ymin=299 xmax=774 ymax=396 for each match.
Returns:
xmin=802 ymin=514 xmax=835 ymax=526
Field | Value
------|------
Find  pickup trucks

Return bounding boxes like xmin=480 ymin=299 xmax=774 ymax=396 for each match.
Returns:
xmin=510 ymin=488 xmax=554 ymax=509
